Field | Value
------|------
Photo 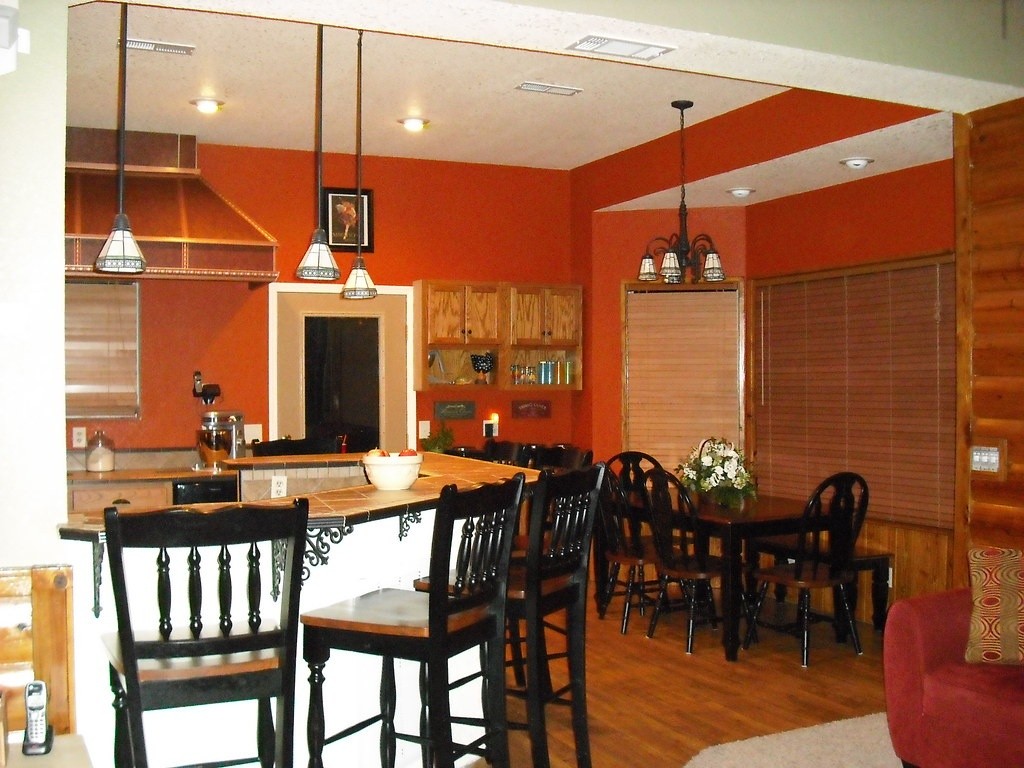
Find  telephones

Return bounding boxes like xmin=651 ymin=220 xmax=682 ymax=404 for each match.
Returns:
xmin=22 ymin=680 xmax=54 ymax=756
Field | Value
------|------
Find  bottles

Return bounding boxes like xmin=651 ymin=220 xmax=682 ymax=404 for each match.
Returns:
xmin=85 ymin=430 xmax=115 ymax=473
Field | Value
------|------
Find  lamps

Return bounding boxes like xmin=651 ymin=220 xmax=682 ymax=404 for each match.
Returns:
xmin=639 ymin=99 xmax=724 ymax=284
xmin=296 ymin=25 xmax=341 ymax=280
xmin=397 ymin=115 xmax=436 ymax=132
xmin=340 ymin=30 xmax=376 ymax=299
xmin=190 ymin=99 xmax=227 ymax=113
xmin=94 ymin=3 xmax=147 ymax=273
xmin=724 ymin=187 xmax=756 ymax=199
xmin=841 ymin=158 xmax=877 ymax=173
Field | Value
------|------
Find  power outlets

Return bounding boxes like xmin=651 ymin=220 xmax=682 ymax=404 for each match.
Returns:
xmin=72 ymin=427 xmax=88 ymax=447
xmin=269 ymin=473 xmax=288 ymax=499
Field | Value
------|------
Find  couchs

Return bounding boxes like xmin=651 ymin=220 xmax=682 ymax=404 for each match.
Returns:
xmin=882 ymin=586 xmax=1024 ymax=768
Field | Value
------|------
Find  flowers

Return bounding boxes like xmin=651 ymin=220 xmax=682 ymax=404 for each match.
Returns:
xmin=673 ymin=437 xmax=760 ymax=512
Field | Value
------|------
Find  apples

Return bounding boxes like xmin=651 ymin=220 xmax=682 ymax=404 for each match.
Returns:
xmin=399 ymin=449 xmax=417 ymax=456
xmin=367 ymin=446 xmax=390 ymax=456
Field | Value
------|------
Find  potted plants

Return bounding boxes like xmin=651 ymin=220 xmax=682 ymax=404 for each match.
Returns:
xmin=420 ymin=424 xmax=456 ymax=454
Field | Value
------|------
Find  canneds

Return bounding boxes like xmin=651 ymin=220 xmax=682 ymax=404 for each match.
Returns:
xmin=512 ymin=364 xmax=536 ymax=384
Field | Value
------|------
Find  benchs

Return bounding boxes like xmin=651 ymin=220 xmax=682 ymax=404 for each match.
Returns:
xmin=742 ymin=537 xmax=890 ymax=628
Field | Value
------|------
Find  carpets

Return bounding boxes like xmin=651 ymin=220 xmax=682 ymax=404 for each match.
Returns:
xmin=680 ymin=711 xmax=901 ymax=768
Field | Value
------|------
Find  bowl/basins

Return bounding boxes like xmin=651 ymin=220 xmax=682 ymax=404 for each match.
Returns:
xmin=362 ymin=452 xmax=423 ymax=491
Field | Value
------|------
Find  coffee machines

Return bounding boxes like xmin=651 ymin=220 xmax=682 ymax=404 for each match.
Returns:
xmin=192 ymin=410 xmax=246 ymax=472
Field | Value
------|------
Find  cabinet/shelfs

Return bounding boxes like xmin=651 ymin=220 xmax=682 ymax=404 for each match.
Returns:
xmin=69 ymin=481 xmax=172 ymax=518
xmin=414 ymin=280 xmax=506 ymax=391
xmin=504 ymin=283 xmax=584 ymax=392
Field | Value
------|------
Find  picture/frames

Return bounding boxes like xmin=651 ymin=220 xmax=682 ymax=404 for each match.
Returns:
xmin=320 ymin=189 xmax=379 ymax=253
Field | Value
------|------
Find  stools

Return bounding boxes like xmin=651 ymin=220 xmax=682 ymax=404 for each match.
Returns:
xmin=102 ymin=437 xmax=596 ymax=768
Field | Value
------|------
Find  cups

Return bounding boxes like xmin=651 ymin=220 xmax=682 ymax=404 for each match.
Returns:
xmin=511 ymin=360 xmax=573 ymax=385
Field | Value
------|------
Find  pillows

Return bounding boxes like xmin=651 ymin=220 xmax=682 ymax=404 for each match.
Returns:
xmin=957 ymin=546 xmax=1023 ymax=666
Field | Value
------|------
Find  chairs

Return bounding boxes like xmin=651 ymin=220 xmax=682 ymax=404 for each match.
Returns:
xmin=593 ymin=451 xmax=903 ymax=671
xmin=0 ymin=564 xmax=94 ymax=768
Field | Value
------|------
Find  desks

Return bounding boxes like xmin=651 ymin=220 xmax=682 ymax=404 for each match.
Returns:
xmin=613 ymin=494 xmax=837 ymax=662
